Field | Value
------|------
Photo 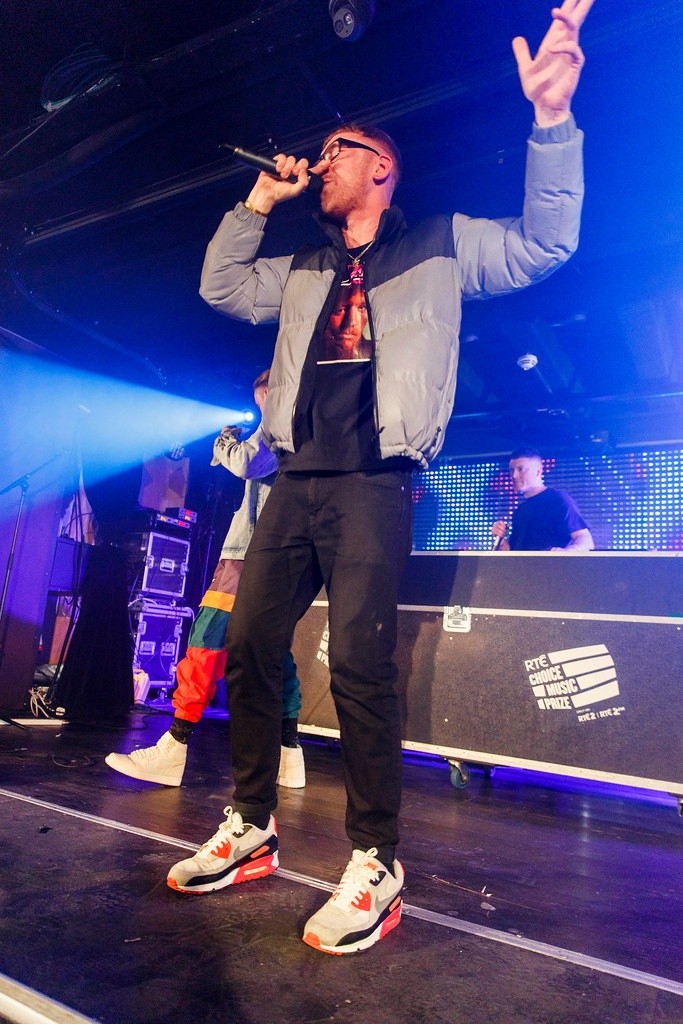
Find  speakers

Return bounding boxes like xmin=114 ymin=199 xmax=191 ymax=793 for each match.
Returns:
xmin=48 ymin=536 xmax=90 ymax=596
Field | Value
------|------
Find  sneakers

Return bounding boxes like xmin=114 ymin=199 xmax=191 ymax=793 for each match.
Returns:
xmin=104 ymin=730 xmax=189 ymax=788
xmin=166 ymin=806 xmax=280 ymax=895
xmin=275 ymin=743 xmax=306 ymax=789
xmin=302 ymin=847 xmax=405 ymax=956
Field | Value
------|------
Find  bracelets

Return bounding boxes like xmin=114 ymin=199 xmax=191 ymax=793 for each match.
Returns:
xmin=243 ymin=199 xmax=269 ymax=218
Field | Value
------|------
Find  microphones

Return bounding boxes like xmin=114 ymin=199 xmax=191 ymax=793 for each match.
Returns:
xmin=218 ymin=143 xmax=324 ymax=195
xmin=493 ymin=521 xmax=511 ymax=551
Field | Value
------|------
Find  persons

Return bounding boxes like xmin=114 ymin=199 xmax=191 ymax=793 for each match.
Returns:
xmin=491 ymin=446 xmax=596 ymax=555
xmin=104 ymin=358 xmax=332 ymax=789
xmin=166 ymin=0 xmax=605 ymax=952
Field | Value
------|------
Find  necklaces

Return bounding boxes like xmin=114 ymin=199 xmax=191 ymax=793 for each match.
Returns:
xmin=342 ymin=237 xmax=376 ymax=265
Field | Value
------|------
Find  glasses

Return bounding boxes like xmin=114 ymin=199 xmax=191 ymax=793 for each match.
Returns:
xmin=312 ymin=137 xmax=381 ymax=168
